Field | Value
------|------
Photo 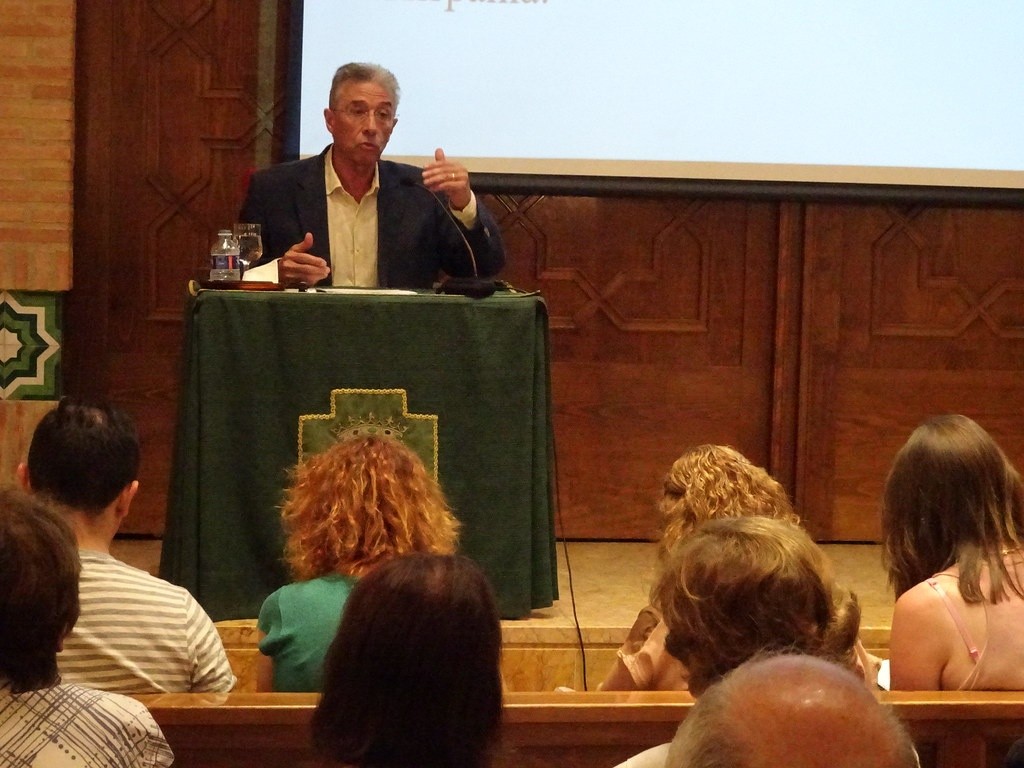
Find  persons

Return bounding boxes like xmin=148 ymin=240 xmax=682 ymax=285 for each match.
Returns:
xmin=310 ymin=553 xmax=502 ymax=768
xmin=611 ymin=516 xmax=920 ymax=768
xmin=255 ymin=436 xmax=465 ymax=692
xmin=664 ymin=648 xmax=918 ymax=768
xmin=889 ymin=414 xmax=1024 ymax=691
xmin=0 ymin=493 xmax=175 ymax=768
xmin=238 ymin=62 xmax=505 ymax=288
xmin=0 ymin=396 xmax=238 ymax=699
xmin=552 ymin=443 xmax=881 ymax=692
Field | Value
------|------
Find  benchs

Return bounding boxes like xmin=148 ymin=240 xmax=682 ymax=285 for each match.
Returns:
xmin=122 ymin=690 xmax=1024 ymax=767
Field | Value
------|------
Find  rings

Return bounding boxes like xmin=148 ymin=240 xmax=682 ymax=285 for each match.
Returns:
xmin=452 ymin=173 xmax=454 ymax=181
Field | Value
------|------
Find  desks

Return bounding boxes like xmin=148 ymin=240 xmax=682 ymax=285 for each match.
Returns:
xmin=158 ymin=284 xmax=561 ymax=622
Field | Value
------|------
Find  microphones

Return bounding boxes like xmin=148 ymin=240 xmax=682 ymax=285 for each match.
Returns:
xmin=400 ymin=176 xmax=497 ymax=299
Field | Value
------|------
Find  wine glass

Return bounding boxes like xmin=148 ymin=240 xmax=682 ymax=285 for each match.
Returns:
xmin=232 ymin=223 xmax=263 ymax=281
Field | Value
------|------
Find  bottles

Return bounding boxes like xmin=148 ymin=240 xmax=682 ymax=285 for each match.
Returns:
xmin=208 ymin=229 xmax=241 ymax=282
